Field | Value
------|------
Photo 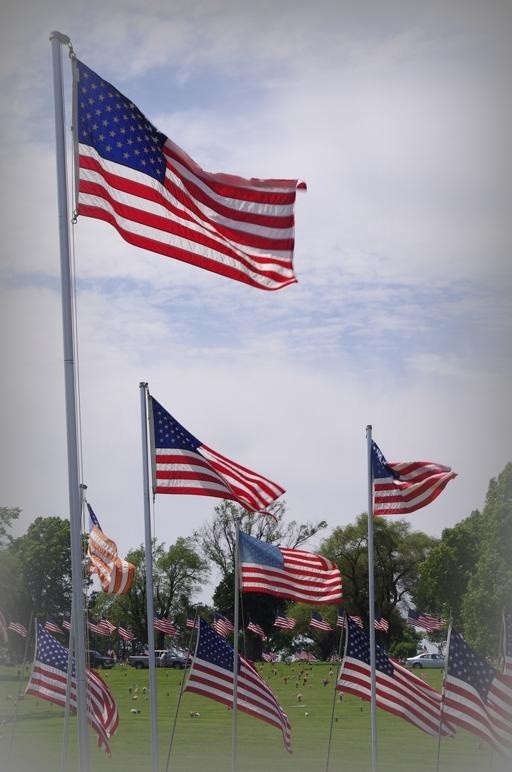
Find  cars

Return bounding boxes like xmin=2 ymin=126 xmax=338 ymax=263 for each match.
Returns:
xmin=405 ymin=651 xmax=447 ymax=669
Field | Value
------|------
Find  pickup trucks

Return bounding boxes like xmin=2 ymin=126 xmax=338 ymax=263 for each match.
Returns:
xmin=127 ymin=647 xmax=192 ymax=670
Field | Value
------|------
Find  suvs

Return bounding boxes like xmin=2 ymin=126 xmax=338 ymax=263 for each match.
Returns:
xmin=66 ymin=647 xmax=115 ymax=670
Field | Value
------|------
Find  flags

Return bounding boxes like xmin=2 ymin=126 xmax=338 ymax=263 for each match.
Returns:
xmin=336 ymin=613 xmax=457 ymax=739
xmin=146 ymin=394 xmax=287 ymax=521
xmin=70 ymin=58 xmax=306 ymax=291
xmin=238 ymin=530 xmax=345 ymax=604
xmin=7 ymin=606 xmax=443 ymax=664
xmin=182 ymin=617 xmax=293 ymax=754
xmin=24 ymin=623 xmax=120 ymax=758
xmin=87 ymin=502 xmax=135 ymax=594
xmin=370 ymin=438 xmax=458 ymax=515
xmin=439 ymin=627 xmax=512 ymax=761
